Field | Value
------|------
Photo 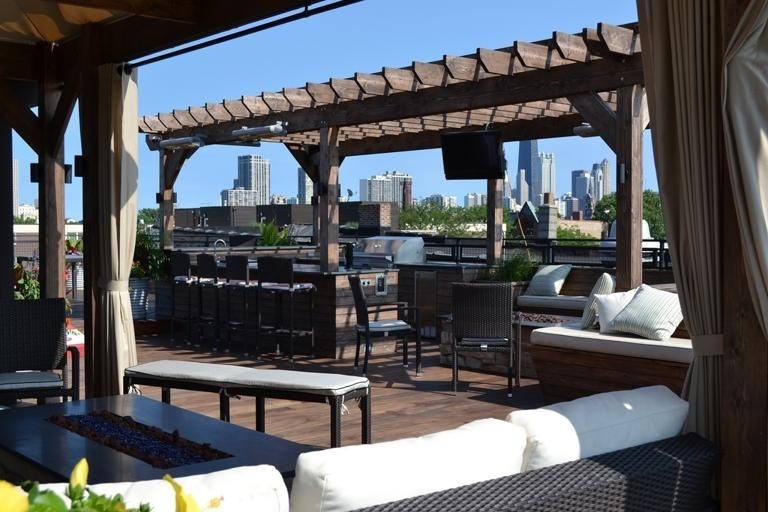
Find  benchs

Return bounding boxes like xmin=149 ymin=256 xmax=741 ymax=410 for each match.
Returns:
xmin=122 ymin=359 xmax=371 ymax=448
xmin=517 ymin=264 xmax=616 ymax=311
xmin=39 ymin=385 xmax=722 ymax=512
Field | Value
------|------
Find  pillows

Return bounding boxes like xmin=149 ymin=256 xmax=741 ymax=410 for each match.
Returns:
xmin=524 ymin=264 xmax=572 ymax=296
xmin=581 ymin=272 xmax=684 ymax=341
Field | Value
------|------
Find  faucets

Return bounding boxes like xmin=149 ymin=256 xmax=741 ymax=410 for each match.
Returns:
xmin=214 ymin=238 xmax=228 ymax=264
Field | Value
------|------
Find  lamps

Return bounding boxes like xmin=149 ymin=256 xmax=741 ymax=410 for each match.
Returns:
xmin=160 ymin=133 xmax=207 ymax=152
xmin=231 ymin=119 xmax=288 ymax=142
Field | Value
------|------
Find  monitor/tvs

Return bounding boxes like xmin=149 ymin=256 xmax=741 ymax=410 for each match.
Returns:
xmin=442 ymin=128 xmax=506 ymax=179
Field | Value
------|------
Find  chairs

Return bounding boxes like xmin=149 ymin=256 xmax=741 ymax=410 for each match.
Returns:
xmin=347 ymin=274 xmax=422 ymax=377
xmin=154 ymin=250 xmax=316 ymax=362
xmin=451 ymin=281 xmax=521 ymax=397
xmin=0 ymin=297 xmax=80 ymax=409
